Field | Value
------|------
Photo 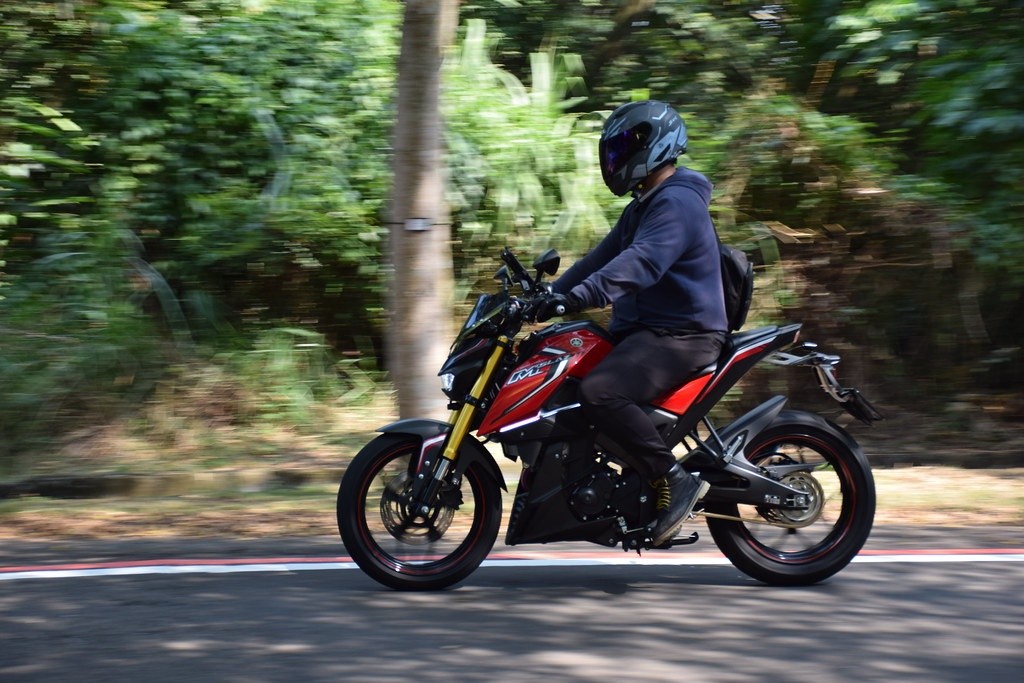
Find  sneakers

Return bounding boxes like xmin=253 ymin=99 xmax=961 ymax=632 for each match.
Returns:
xmin=650 ymin=464 xmax=712 ymax=546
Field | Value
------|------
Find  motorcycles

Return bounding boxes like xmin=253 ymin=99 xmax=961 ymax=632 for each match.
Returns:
xmin=334 ymin=244 xmax=885 ymax=592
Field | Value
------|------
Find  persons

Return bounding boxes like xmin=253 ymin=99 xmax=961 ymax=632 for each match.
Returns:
xmin=520 ymin=99 xmax=729 ymax=547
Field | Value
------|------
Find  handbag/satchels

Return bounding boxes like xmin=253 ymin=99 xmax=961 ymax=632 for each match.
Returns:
xmin=720 ymin=245 xmax=754 ymax=331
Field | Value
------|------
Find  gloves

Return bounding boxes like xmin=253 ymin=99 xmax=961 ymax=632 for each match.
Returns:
xmin=534 ymin=282 xmax=551 ymax=298
xmin=536 ymin=293 xmax=582 ymax=322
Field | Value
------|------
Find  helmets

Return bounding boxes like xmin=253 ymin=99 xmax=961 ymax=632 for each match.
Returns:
xmin=599 ymin=100 xmax=688 ymax=197
